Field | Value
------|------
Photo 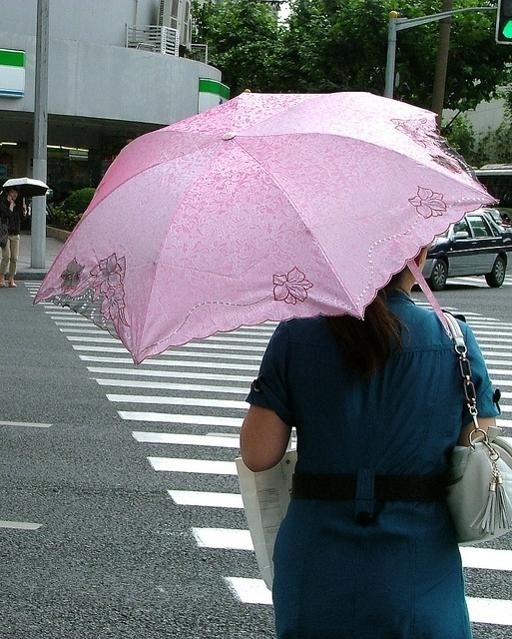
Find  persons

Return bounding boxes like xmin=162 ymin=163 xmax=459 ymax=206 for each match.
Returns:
xmin=239 ymin=246 xmax=502 ymax=639
xmin=0 ymin=188 xmax=26 ymax=288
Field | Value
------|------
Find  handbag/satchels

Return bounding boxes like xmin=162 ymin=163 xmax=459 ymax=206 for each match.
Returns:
xmin=445 ymin=426 xmax=512 ymax=545
xmin=0 ymin=221 xmax=8 ymax=248
xmin=234 ymin=437 xmax=298 ymax=591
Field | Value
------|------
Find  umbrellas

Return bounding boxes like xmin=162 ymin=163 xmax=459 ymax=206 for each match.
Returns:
xmin=33 ymin=91 xmax=500 ymax=369
xmin=3 ymin=177 xmax=49 ymax=204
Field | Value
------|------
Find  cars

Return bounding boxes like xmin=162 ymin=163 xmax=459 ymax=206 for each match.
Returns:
xmin=412 ymin=207 xmax=512 ymax=295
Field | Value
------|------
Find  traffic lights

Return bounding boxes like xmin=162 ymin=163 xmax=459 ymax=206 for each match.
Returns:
xmin=495 ymin=0 xmax=512 ymax=46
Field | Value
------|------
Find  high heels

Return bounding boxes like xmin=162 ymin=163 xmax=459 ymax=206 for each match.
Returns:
xmin=0 ymin=276 xmax=17 ymax=287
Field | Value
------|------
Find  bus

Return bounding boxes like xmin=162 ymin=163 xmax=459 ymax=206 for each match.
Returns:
xmin=471 ymin=162 xmax=512 ymax=224
